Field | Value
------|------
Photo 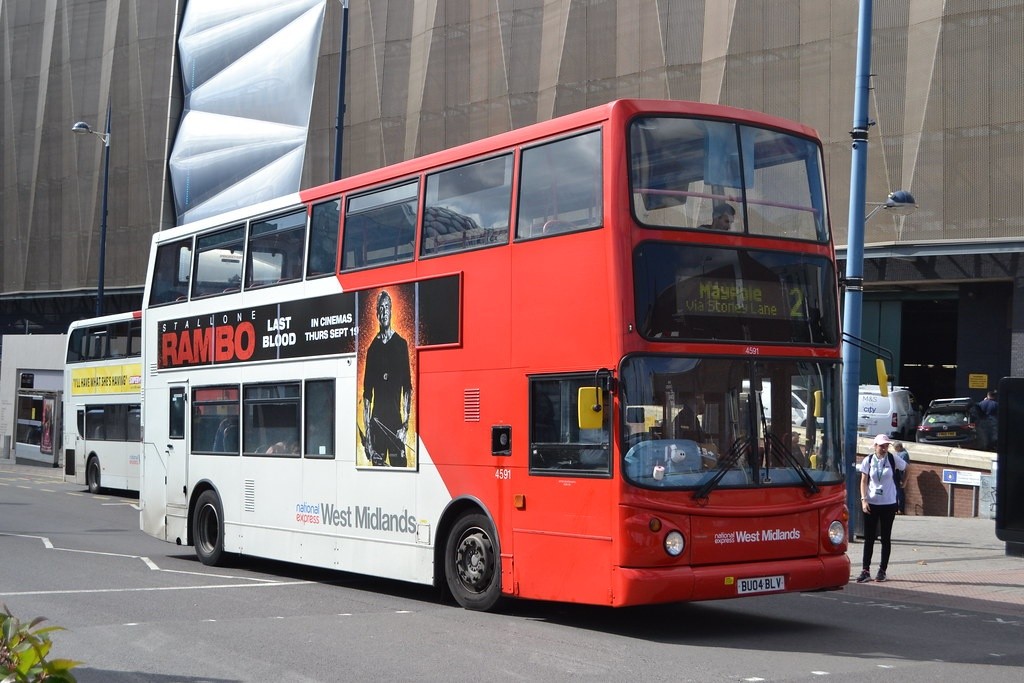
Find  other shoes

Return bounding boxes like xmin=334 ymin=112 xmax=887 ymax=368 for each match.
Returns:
xmin=895 ymin=511 xmax=905 ymax=515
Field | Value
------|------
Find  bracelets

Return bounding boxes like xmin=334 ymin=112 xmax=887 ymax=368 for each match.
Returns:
xmin=861 ymin=496 xmax=867 ymax=500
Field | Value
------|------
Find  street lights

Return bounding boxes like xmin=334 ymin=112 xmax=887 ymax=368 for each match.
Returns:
xmin=72 ymin=98 xmax=112 ymax=358
xmin=840 ymin=189 xmax=918 ymax=541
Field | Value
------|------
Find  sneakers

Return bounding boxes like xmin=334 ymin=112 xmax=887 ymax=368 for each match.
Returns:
xmin=874 ymin=568 xmax=886 ymax=582
xmin=857 ymin=571 xmax=871 ymax=583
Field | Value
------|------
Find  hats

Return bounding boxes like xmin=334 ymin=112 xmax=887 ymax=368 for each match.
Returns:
xmin=792 ymin=432 xmax=801 ymax=438
xmin=873 ymin=434 xmax=892 ymax=445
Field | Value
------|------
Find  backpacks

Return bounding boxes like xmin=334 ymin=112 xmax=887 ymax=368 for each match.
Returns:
xmin=867 ymin=451 xmax=900 ymax=493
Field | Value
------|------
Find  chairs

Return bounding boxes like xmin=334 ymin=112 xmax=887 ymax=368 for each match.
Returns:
xmin=176 ymin=220 xmax=577 ymax=300
xmin=213 ymin=417 xmax=253 ymax=452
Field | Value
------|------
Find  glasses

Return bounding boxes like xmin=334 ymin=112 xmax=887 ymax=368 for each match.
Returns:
xmin=292 ymin=450 xmax=301 ymax=454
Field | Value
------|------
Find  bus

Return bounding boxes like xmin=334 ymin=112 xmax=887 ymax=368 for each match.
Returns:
xmin=62 ymin=310 xmax=142 ymax=494
xmin=140 ymin=99 xmax=897 ymax=613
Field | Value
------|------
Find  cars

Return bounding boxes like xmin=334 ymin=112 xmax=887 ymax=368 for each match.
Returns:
xmin=916 ymin=397 xmax=979 ymax=449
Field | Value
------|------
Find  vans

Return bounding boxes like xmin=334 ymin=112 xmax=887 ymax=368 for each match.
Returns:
xmin=857 ymin=384 xmax=924 ymax=442
xmin=742 ymin=379 xmax=824 ymax=429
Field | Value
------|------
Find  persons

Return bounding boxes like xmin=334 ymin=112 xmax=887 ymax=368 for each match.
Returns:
xmin=978 ymin=391 xmax=999 ymax=452
xmin=362 ymin=290 xmax=412 ymax=467
xmin=856 ymin=434 xmax=912 ymax=583
xmin=266 ymin=436 xmax=300 ymax=455
xmin=698 ymin=203 xmax=735 ymax=231
xmin=675 ymin=387 xmax=732 ymax=462
xmin=214 ymin=406 xmax=239 ymax=452
xmin=892 ymin=441 xmax=909 ymax=515
xmin=738 ymin=425 xmax=819 ymax=470
